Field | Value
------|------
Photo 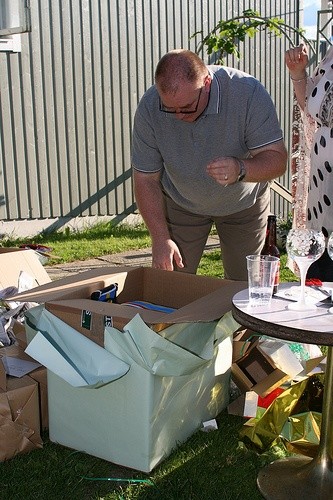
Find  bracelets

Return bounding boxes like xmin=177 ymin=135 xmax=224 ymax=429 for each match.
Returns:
xmin=291 ymin=72 xmax=308 ymax=85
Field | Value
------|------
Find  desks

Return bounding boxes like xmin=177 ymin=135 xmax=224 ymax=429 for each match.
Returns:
xmin=232 ymin=282 xmax=333 ymax=500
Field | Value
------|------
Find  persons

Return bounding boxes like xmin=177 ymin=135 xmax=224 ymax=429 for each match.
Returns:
xmin=132 ymin=50 xmax=289 ymax=282
xmin=285 ymin=38 xmax=333 ymax=286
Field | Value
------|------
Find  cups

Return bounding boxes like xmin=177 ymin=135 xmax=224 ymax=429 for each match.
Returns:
xmin=246 ymin=255 xmax=280 ymax=307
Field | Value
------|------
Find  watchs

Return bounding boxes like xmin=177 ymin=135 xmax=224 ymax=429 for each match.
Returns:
xmin=237 ymin=159 xmax=246 ymax=182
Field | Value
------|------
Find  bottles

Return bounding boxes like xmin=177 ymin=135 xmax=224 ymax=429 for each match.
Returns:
xmin=260 ymin=214 xmax=280 ymax=294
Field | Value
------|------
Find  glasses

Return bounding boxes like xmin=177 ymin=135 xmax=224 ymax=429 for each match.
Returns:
xmin=159 ymin=84 xmax=204 ymax=114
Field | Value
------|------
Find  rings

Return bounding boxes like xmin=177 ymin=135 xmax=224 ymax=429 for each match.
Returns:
xmin=224 ymin=173 xmax=228 ymax=181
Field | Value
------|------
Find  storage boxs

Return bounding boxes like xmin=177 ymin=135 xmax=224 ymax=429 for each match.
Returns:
xmin=0 ymin=248 xmax=325 ymax=474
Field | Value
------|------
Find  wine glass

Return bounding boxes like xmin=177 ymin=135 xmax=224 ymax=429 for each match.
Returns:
xmin=326 ymin=232 xmax=333 ymax=314
xmin=285 ymin=228 xmax=325 ymax=310
